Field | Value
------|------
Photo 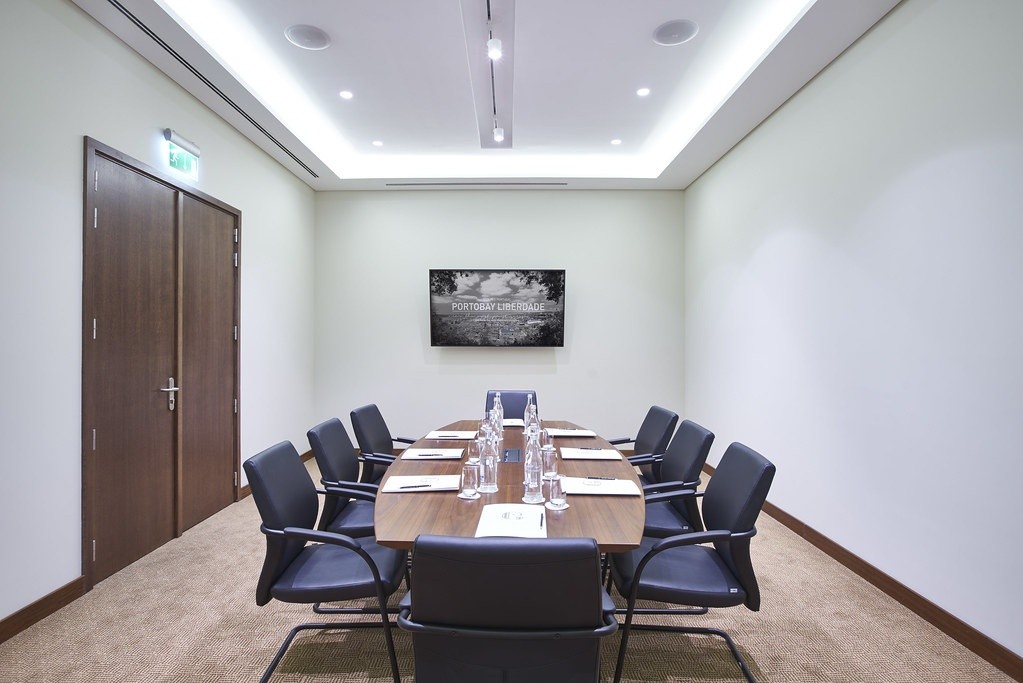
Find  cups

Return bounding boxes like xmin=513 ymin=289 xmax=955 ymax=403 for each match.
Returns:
xmin=461 ymin=464 xmax=478 ymax=497
xmin=549 ymin=474 xmax=567 ymax=508
xmin=544 ymin=453 xmax=558 ymax=478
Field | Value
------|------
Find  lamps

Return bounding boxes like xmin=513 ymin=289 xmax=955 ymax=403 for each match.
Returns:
xmin=493 ymin=127 xmax=504 ymax=143
xmin=487 ymin=38 xmax=502 ymax=61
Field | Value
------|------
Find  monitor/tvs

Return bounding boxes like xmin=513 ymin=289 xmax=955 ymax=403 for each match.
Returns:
xmin=429 ymin=269 xmax=565 ymax=348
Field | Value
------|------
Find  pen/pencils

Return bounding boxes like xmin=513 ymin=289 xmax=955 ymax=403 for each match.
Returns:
xmin=419 ymin=454 xmax=443 ymax=456
xmin=541 ymin=513 xmax=543 ymax=527
xmin=439 ymin=436 xmax=458 ymax=437
xmin=400 ymin=485 xmax=431 ymax=488
xmin=580 ymin=448 xmax=602 ymax=450
xmin=587 ymin=477 xmax=615 ymax=480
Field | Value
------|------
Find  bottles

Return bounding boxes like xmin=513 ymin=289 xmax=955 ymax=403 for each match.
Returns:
xmin=478 ymin=391 xmax=546 ymax=502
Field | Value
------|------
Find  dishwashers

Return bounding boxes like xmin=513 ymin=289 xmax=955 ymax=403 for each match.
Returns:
xmin=466 ymin=439 xmax=480 ymax=463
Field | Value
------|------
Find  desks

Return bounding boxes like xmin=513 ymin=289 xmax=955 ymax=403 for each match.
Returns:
xmin=373 ymin=420 xmax=647 ymax=554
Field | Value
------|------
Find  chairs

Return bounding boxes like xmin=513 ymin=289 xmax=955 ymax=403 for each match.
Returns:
xmin=397 ymin=533 xmax=620 ymax=683
xmin=243 ymin=403 xmax=412 ymax=683
xmin=600 ymin=405 xmax=776 ymax=683
xmin=487 ymin=389 xmax=539 ymax=422
